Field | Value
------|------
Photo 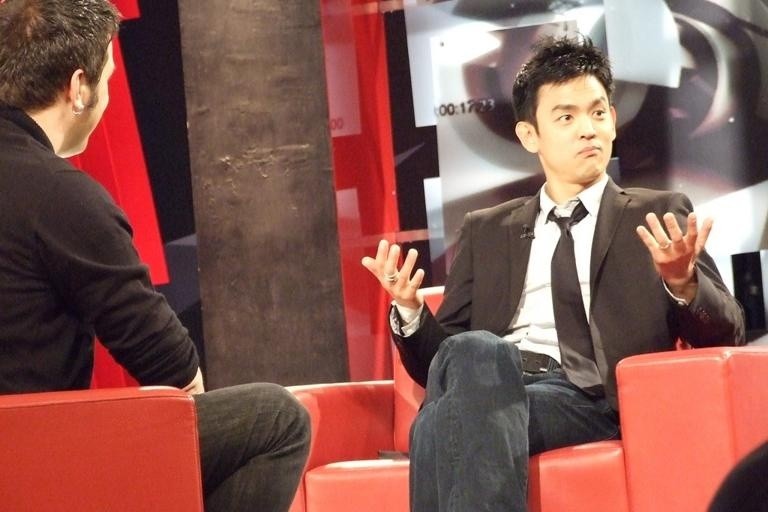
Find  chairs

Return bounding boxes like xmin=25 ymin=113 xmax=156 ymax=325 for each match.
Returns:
xmin=282 ymin=281 xmax=768 ymax=512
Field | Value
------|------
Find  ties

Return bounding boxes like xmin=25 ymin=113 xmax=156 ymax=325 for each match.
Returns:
xmin=547 ymin=202 xmax=606 ymax=403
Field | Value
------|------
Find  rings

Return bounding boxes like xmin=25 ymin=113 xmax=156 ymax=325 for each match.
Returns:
xmin=661 ymin=245 xmax=670 ymax=250
xmin=383 ymin=275 xmax=398 ymax=282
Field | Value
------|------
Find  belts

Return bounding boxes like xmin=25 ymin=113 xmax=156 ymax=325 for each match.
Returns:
xmin=516 ymin=350 xmax=550 ymax=374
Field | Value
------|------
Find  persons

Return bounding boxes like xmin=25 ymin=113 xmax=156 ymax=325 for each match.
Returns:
xmin=358 ymin=30 xmax=746 ymax=512
xmin=0 ymin=0 xmax=313 ymax=512
